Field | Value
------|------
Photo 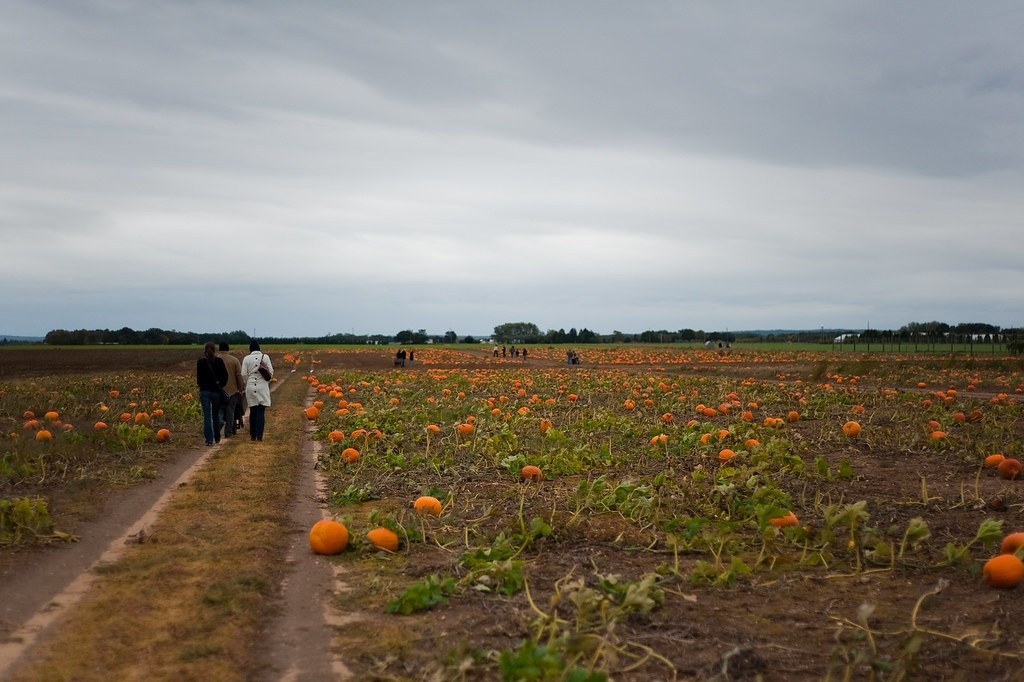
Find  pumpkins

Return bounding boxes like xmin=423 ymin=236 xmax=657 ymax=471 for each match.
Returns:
xmin=24 ymin=349 xmax=1024 ymax=587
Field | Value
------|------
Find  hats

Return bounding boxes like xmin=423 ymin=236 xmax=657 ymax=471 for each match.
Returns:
xmin=219 ymin=342 xmax=229 ymax=351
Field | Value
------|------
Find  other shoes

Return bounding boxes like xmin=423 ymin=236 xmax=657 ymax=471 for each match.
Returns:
xmin=257 ymin=436 xmax=262 ymax=441
xmin=205 ymin=440 xmax=213 ymax=446
xmin=215 ymin=440 xmax=220 ymax=443
xmin=225 ymin=433 xmax=231 ymax=438
xmin=232 ymin=428 xmax=236 ymax=435
xmin=251 ymin=435 xmax=256 ymax=441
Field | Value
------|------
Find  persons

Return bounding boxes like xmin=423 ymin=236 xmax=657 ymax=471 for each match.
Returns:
xmin=197 ymin=341 xmax=243 ymax=445
xmin=494 ymin=344 xmax=527 ymax=361
xmin=241 ymin=342 xmax=273 ymax=441
xmin=567 ymin=349 xmax=578 ymax=365
xmin=396 ymin=349 xmax=414 ymax=367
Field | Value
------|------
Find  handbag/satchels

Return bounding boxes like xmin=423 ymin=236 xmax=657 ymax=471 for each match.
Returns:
xmin=219 ymin=388 xmax=229 ymax=409
xmin=258 ymin=353 xmax=271 ymax=381
